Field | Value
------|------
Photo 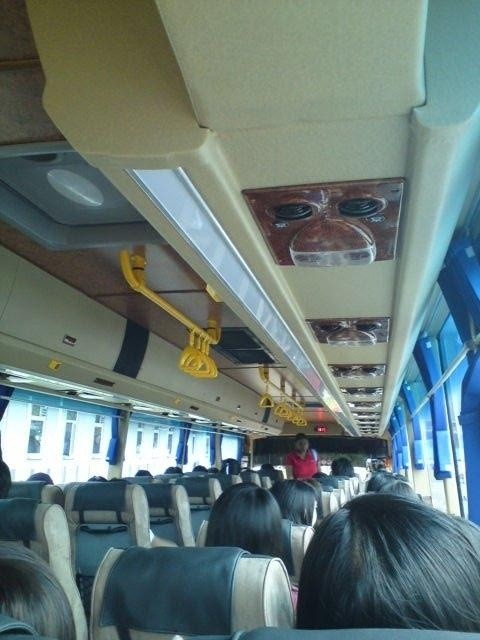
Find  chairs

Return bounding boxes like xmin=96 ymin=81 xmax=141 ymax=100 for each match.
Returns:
xmin=0 ymin=464 xmax=479 ymax=638
